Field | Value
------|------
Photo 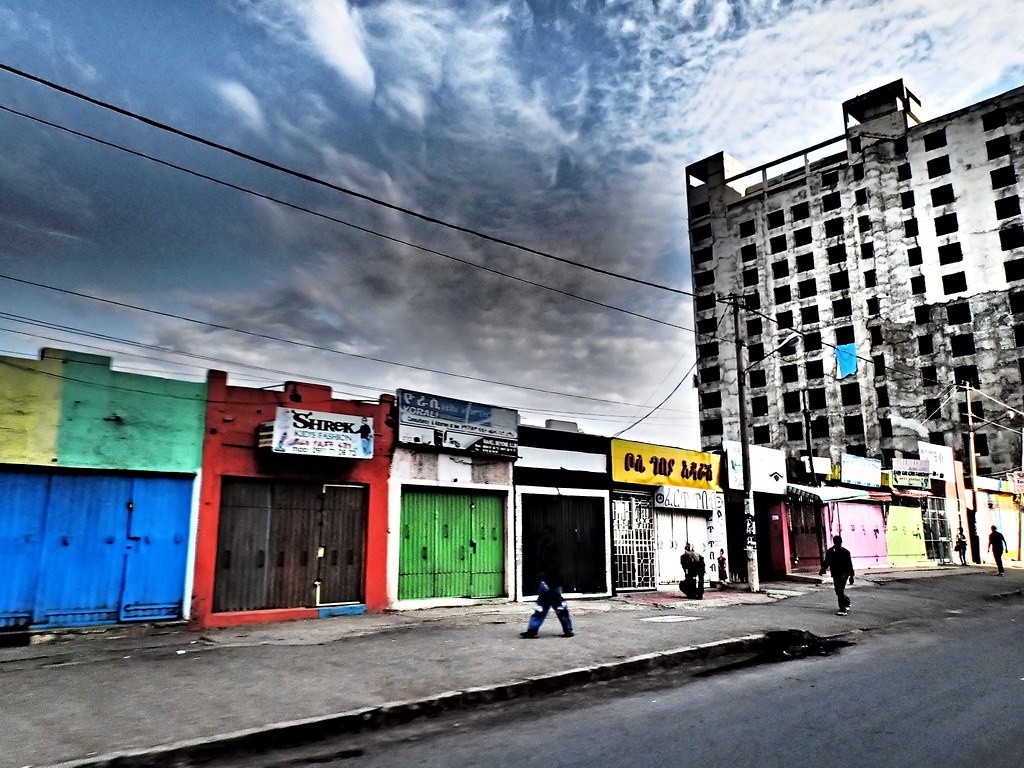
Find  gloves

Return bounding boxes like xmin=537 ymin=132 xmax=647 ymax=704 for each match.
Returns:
xmin=820 ymin=567 xmax=826 ymax=574
xmin=849 ymin=578 xmax=854 ymax=585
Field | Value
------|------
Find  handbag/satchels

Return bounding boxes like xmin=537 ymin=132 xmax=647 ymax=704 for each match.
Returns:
xmin=954 ymin=542 xmax=960 ymax=551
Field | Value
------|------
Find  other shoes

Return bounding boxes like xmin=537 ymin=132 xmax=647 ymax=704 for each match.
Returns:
xmin=962 ymin=563 xmax=966 ymax=566
xmin=836 ymin=609 xmax=847 ymax=615
xmin=563 ymin=632 xmax=574 ymax=637
xmin=520 ymin=631 xmax=539 ymax=638
xmin=994 ymin=573 xmax=1005 ymax=576
xmin=845 ymin=602 xmax=850 ymax=611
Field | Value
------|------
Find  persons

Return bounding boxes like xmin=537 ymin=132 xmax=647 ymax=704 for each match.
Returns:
xmin=680 ymin=542 xmax=705 ymax=600
xmin=717 ymin=549 xmax=728 ymax=582
xmin=520 ymin=523 xmax=575 ymax=639
xmin=954 ymin=527 xmax=970 ymax=566
xmin=818 ymin=535 xmax=854 ymax=616
xmin=988 ymin=525 xmax=1008 ymax=578
